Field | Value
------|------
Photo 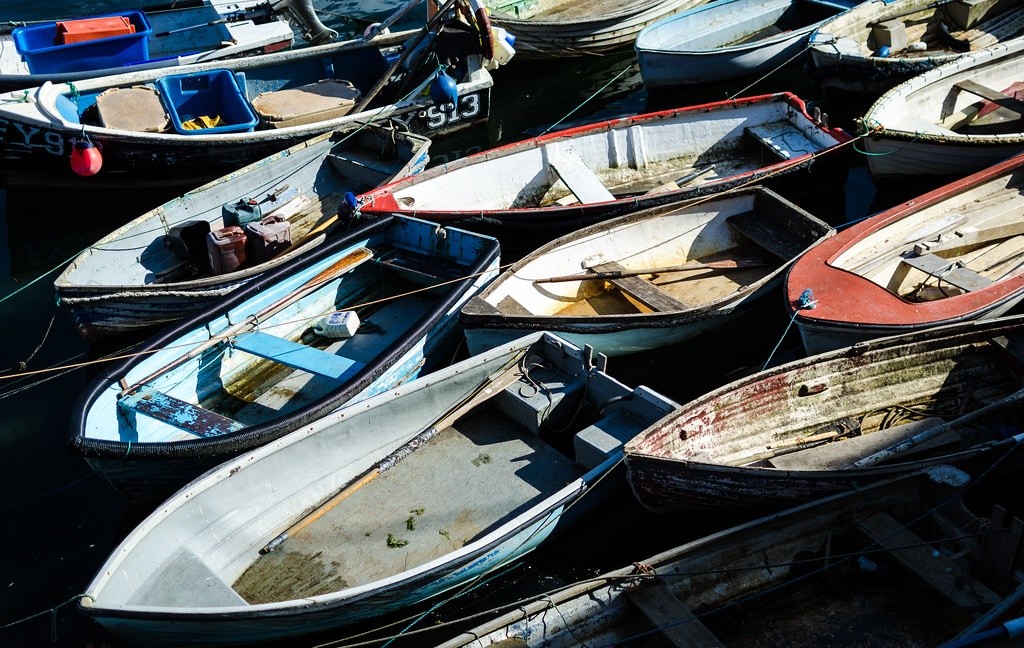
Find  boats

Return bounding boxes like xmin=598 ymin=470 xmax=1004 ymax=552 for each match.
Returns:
xmin=459 ymin=184 xmax=837 ymax=380
xmin=808 ymin=0 xmax=1024 ymax=84
xmin=79 ymin=331 xmax=683 ymax=648
xmin=435 ymin=433 xmax=1024 ymax=648
xmin=338 ymin=91 xmax=873 ymax=261
xmin=623 ymin=313 xmax=1024 ymax=516
xmin=634 ymin=0 xmax=873 ymax=89
xmin=853 ymin=36 xmax=1024 ymax=174
xmin=72 ymin=212 xmax=502 ymax=500
xmin=54 ymin=121 xmax=432 ymax=349
xmin=489 ymin=0 xmax=712 ymax=59
xmin=0 ymin=0 xmax=495 ymax=176
xmin=786 ymin=153 xmax=1024 ymax=356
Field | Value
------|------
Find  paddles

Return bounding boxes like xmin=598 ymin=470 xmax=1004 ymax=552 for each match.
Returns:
xmin=115 ymin=246 xmax=374 ymax=399
xmin=351 ymin=0 xmax=457 ymax=116
xmin=646 ymin=163 xmax=717 ymax=197
xmin=534 ymin=254 xmax=770 ymax=287
xmin=265 ymin=353 xmax=546 ymax=554
xmin=852 ymin=388 xmax=1023 ymax=470
xmin=273 ymin=170 xmax=400 ymax=259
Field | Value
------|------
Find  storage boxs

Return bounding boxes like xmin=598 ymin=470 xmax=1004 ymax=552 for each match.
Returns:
xmin=12 ymin=9 xmax=153 ymax=74
xmin=156 ymin=68 xmax=260 ymax=134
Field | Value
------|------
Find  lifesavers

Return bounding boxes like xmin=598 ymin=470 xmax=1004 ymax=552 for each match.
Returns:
xmin=457 ymin=0 xmax=494 ymax=61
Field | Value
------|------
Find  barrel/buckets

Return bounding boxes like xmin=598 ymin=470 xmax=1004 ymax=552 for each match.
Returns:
xmin=314 ymin=311 xmax=361 ymax=337
xmin=206 ymin=197 xmax=293 ymax=276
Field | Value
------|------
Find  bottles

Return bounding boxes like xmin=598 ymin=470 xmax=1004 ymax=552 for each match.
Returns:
xmin=483 ymin=26 xmax=516 ymax=69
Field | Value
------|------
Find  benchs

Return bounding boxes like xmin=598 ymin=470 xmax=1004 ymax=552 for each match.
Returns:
xmin=859 ymin=512 xmax=1003 ymax=619
xmin=545 ymin=140 xmax=616 ymax=204
xmin=768 ymin=417 xmax=962 ymax=471
xmin=811 ymin=0 xmax=857 ymax=10
xmin=726 ymin=210 xmax=808 ymax=264
xmin=369 ymin=243 xmax=472 ymax=283
xmin=487 ymin=636 xmax=529 ymax=648
xmin=329 ymin=149 xmax=406 ymax=174
xmin=118 ymin=385 xmax=250 ymax=438
xmin=746 ymin=120 xmax=823 ymax=161
xmin=903 ymin=253 xmax=995 ymax=292
xmin=591 ymin=261 xmax=690 ymax=313
xmin=626 ymin=579 xmax=726 ymax=648
xmin=955 ymin=80 xmax=1024 ymax=114
xmin=224 ymin=329 xmax=367 ymax=382
